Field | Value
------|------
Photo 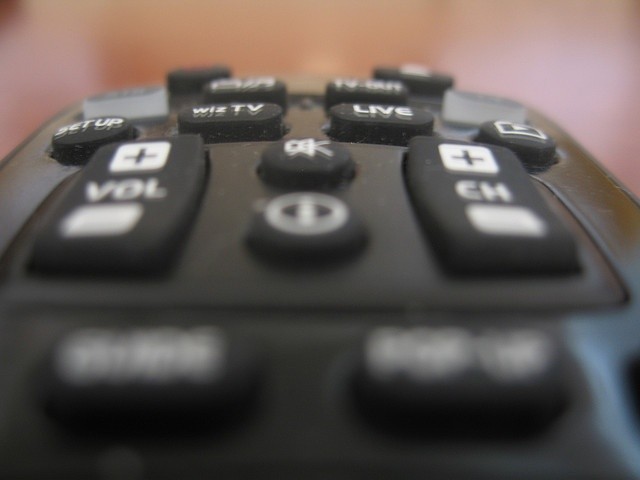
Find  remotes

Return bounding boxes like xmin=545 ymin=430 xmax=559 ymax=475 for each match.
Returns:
xmin=1 ymin=64 xmax=639 ymax=480
